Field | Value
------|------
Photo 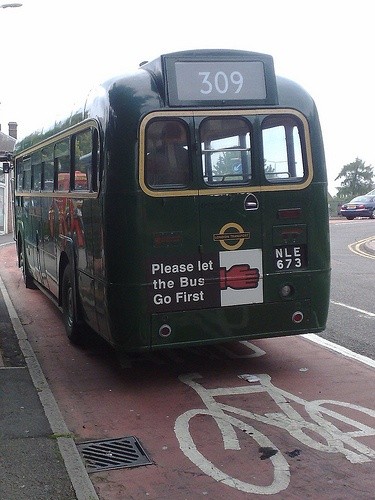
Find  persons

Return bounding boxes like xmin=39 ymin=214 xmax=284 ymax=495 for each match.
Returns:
xmin=155 ymin=122 xmax=190 ymax=184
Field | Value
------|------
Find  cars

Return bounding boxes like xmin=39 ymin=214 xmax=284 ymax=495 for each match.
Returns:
xmin=340 ymin=194 xmax=375 ymax=220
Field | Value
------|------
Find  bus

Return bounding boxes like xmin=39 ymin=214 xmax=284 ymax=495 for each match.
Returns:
xmin=3 ymin=49 xmax=331 ymax=364
